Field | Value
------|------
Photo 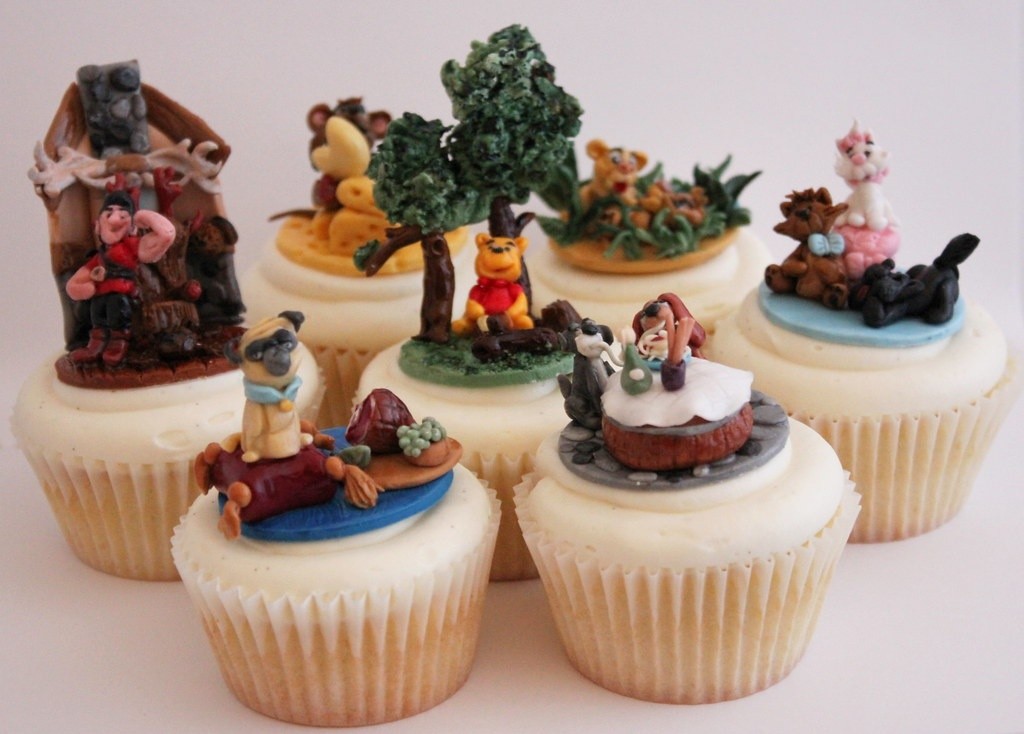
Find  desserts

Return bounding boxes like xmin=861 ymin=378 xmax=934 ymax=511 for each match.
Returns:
xmin=11 ymin=21 xmax=1024 ymax=727
